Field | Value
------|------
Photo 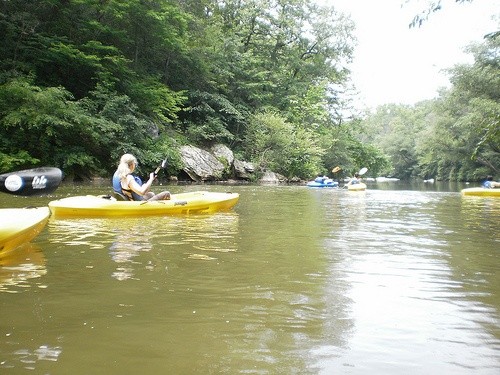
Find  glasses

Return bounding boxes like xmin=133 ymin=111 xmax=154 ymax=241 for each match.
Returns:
xmin=129 ymin=162 xmax=138 ymax=167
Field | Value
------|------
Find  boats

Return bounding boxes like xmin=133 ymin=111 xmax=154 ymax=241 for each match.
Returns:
xmin=48 ymin=190 xmax=240 ymax=216
xmin=0 ymin=206 xmax=50 ymax=258
xmin=306 ymin=180 xmax=367 ymax=190
xmin=463 ymin=188 xmax=500 ymax=197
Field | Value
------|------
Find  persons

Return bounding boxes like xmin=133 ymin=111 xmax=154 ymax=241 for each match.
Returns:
xmin=349 ymin=173 xmax=360 ymax=185
xmin=112 ymin=154 xmax=169 ymax=202
xmin=484 ymin=176 xmax=499 ymax=189
